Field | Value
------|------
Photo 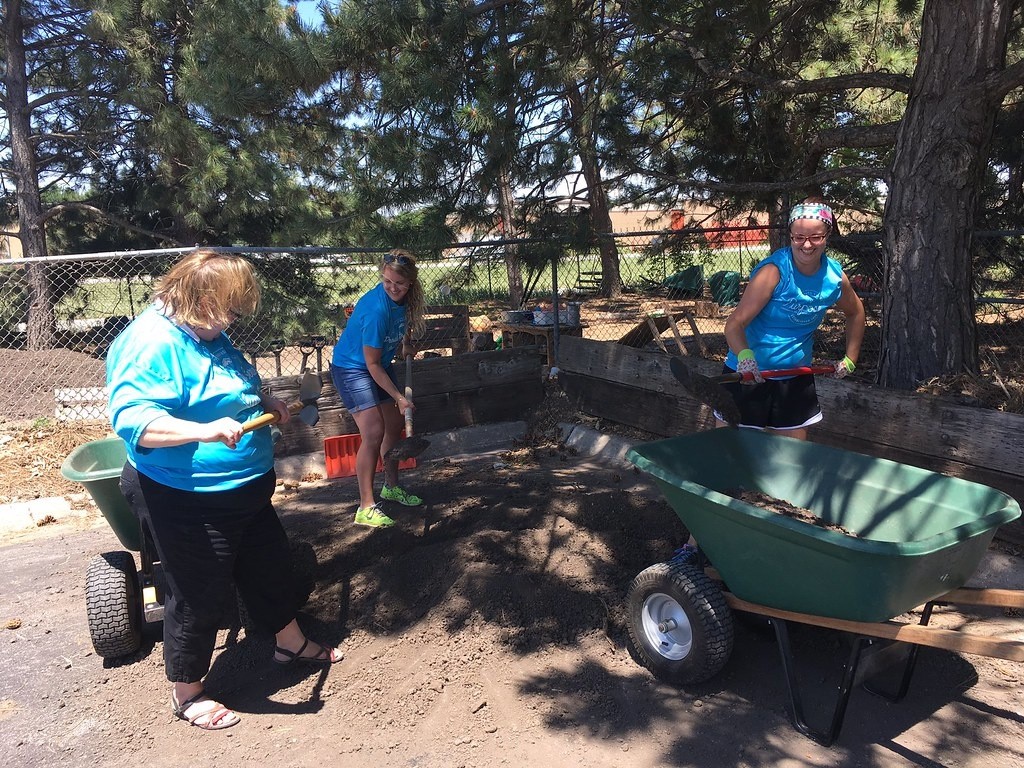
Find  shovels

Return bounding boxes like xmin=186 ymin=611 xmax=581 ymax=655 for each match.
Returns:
xmin=240 ymin=372 xmax=323 ymax=440
xmin=384 ymin=354 xmax=430 ymax=462
xmin=669 ymin=355 xmax=836 ymax=409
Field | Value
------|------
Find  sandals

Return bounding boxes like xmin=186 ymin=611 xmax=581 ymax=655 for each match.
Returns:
xmin=273 ymin=638 xmax=343 ymax=665
xmin=172 ymin=688 xmax=240 ymax=730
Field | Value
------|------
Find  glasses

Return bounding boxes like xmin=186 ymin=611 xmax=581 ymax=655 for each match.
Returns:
xmin=383 ymin=253 xmax=413 ymax=267
xmin=790 ymin=228 xmax=831 ymax=245
xmin=227 ymin=307 xmax=243 ymax=319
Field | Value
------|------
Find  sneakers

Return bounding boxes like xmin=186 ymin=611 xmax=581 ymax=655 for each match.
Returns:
xmin=671 ymin=543 xmax=702 ymax=565
xmin=353 ymin=501 xmax=396 ymax=529
xmin=380 ymin=484 xmax=422 ymax=506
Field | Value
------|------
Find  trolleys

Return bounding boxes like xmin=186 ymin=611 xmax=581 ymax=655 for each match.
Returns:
xmin=60 ymin=436 xmax=259 ymax=658
xmin=623 ymin=424 xmax=1024 ymax=748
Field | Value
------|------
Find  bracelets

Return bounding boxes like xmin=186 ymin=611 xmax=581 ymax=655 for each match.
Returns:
xmin=395 ymin=396 xmax=403 ymax=407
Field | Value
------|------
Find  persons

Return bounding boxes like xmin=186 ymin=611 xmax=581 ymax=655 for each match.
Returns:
xmin=106 ymin=247 xmax=343 ymax=729
xmin=332 ymin=249 xmax=425 ymax=529
xmin=671 ymin=197 xmax=866 ymax=563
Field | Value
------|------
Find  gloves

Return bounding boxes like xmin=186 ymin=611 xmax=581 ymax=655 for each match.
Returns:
xmin=736 ymin=349 xmax=765 ymax=386
xmin=825 ymin=354 xmax=855 ymax=380
